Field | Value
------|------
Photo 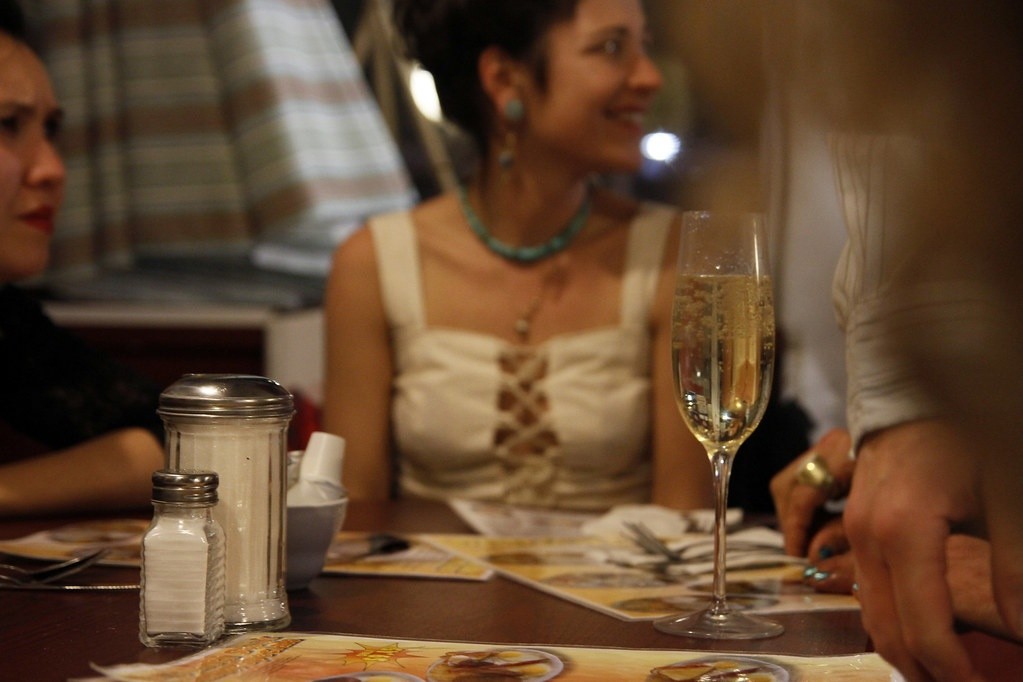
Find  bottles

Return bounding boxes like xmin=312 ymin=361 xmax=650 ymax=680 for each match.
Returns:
xmin=140 ymin=467 xmax=228 ymax=645
xmin=156 ymin=370 xmax=291 ymax=634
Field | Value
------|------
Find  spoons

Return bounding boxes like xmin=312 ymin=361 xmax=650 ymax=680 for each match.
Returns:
xmin=654 ymin=556 xmax=805 ymax=583
xmin=0 ymin=546 xmax=109 ymax=584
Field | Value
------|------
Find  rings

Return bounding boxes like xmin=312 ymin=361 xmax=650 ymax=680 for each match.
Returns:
xmin=801 ymin=454 xmax=844 ymax=502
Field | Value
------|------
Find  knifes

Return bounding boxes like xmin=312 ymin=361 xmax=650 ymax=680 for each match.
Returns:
xmin=0 ymin=580 xmax=139 ymax=591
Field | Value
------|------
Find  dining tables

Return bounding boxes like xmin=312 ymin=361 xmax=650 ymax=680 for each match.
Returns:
xmin=0 ymin=500 xmax=1023 ymax=681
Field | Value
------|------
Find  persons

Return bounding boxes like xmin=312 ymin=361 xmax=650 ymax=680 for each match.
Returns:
xmin=772 ymin=423 xmax=1016 ymax=655
xmin=0 ymin=8 xmax=169 ymax=519
xmin=800 ymin=2 xmax=1023 ymax=681
xmin=320 ymin=0 xmax=731 ymax=515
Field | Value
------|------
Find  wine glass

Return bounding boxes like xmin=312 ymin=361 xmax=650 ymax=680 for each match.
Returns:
xmin=654 ymin=209 xmax=784 ymax=638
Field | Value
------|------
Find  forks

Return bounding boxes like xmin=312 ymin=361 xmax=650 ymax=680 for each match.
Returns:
xmin=618 ymin=516 xmax=789 ymax=558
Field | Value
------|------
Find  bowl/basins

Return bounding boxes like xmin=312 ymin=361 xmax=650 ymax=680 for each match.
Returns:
xmin=285 ymin=497 xmax=347 ymax=590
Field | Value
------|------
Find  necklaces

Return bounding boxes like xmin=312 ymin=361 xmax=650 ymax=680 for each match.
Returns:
xmin=454 ymin=175 xmax=604 ymax=264
xmin=504 ymin=261 xmax=557 ymax=336
